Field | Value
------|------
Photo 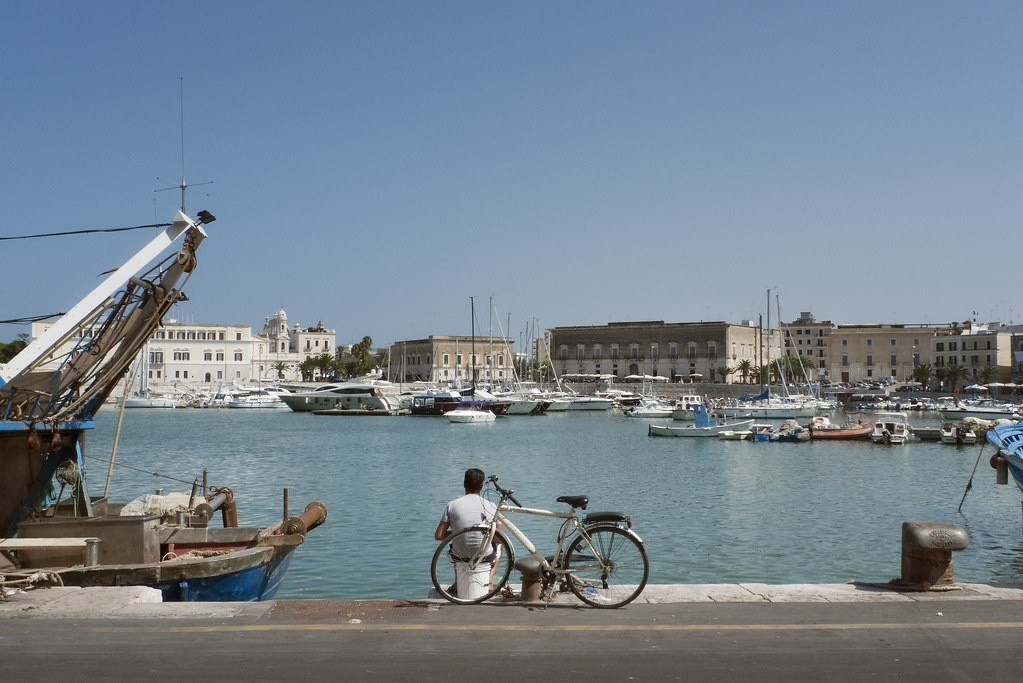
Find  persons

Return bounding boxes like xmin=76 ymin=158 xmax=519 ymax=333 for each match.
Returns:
xmin=550 ymin=377 xmax=599 ymax=383
xmin=435 ymin=468 xmax=502 ymax=594
xmin=679 ymin=377 xmax=702 ymax=383
xmin=808 ymin=419 xmax=815 ymax=436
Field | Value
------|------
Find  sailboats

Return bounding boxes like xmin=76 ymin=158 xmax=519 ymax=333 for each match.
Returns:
xmin=116 ymin=292 xmax=612 ymax=414
xmin=708 ymin=287 xmax=844 ymax=418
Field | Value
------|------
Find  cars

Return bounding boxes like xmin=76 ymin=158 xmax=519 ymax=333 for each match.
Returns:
xmin=841 ymin=383 xmax=851 ymax=388
xmin=851 ymin=377 xmax=898 ymax=389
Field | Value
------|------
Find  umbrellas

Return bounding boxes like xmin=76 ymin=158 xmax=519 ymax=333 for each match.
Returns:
xmin=625 ymin=375 xmax=669 ymax=380
xmin=559 ymin=374 xmax=618 ymax=378
xmin=690 ymin=373 xmax=703 ymax=377
xmin=964 ymin=382 xmax=1023 ymax=399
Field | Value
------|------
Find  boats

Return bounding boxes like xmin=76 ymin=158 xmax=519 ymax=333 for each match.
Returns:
xmin=50 ymin=464 xmax=329 ymax=607
xmin=717 ymin=428 xmax=753 ymax=441
xmin=672 ymin=394 xmax=704 ymax=423
xmin=940 ymin=423 xmax=978 ymax=444
xmin=593 ymin=383 xmax=674 ymax=419
xmin=747 ymin=416 xmax=874 ymax=442
xmin=444 ymin=408 xmax=496 ymax=424
xmin=985 ymin=420 xmax=1023 ymax=500
xmin=648 ymin=402 xmax=756 ymax=437
xmin=870 ymin=411 xmax=912 ymax=445
xmin=856 ymin=394 xmax=1023 ymax=410
xmin=937 ymin=400 xmax=1015 ymax=421
xmin=1 ymin=202 xmax=217 ymax=542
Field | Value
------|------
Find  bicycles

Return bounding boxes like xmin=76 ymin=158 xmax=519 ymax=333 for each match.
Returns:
xmin=430 ymin=471 xmax=651 ymax=610
